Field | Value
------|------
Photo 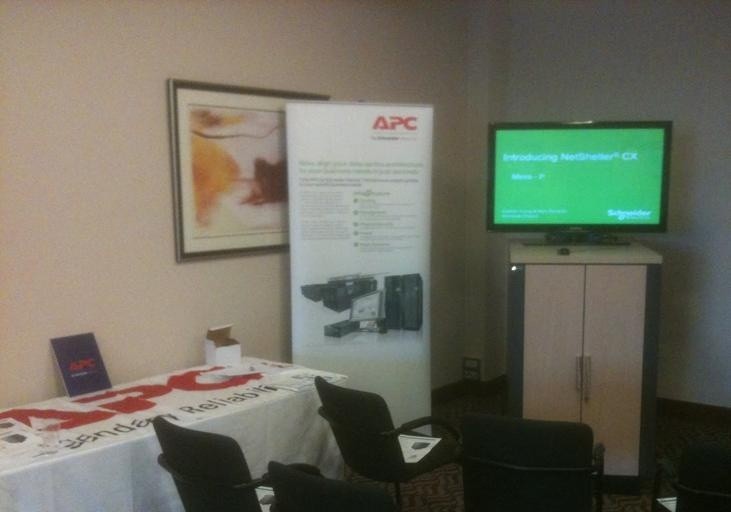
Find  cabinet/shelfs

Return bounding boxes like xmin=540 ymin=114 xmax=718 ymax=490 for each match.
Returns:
xmin=507 ymin=238 xmax=663 ymax=494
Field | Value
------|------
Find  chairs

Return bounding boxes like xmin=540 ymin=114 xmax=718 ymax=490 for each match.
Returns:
xmin=267 ymin=462 xmax=394 ymax=511
xmin=153 ymin=415 xmax=324 ymax=510
xmin=461 ymin=414 xmax=604 ymax=510
xmin=650 ymin=444 xmax=731 ymax=511
xmin=315 ymin=377 xmax=480 ymax=511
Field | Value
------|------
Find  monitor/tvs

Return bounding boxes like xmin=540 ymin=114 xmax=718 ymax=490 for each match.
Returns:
xmin=349 ymin=290 xmax=386 ymax=332
xmin=484 ymin=120 xmax=674 ymax=247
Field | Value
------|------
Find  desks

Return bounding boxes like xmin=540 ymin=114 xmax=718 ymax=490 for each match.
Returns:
xmin=0 ymin=356 xmax=348 ymax=512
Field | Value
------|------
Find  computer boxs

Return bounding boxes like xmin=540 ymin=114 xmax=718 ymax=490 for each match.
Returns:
xmin=384 ymin=273 xmax=423 ymax=330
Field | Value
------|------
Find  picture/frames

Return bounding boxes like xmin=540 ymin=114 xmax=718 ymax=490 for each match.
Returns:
xmin=168 ymin=79 xmax=330 ymax=263
